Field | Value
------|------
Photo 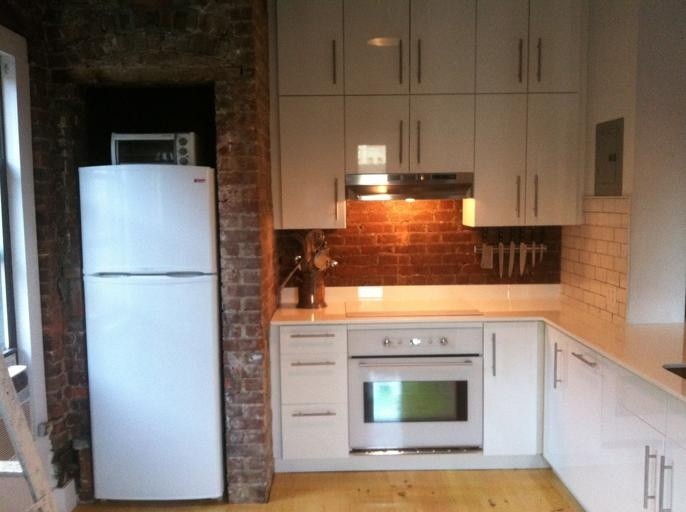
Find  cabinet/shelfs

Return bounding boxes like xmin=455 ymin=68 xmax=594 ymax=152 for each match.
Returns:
xmin=483 ymin=320 xmax=539 ymax=469
xmin=344 ymin=2 xmax=476 ymax=95
xmin=345 ymin=94 xmax=475 ymax=175
xmin=277 ymin=0 xmax=345 ymax=94
xmin=271 ymin=320 xmax=348 ymax=472
xmin=346 ymin=323 xmax=482 ymax=472
xmin=538 ymin=319 xmax=686 ymax=512
xmin=279 ymin=96 xmax=346 ymax=229
xmin=478 ymin=2 xmax=591 ymax=94
xmin=462 ymin=94 xmax=589 ymax=227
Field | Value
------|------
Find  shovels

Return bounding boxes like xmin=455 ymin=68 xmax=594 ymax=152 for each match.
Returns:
xmin=302 ymin=230 xmax=339 ymax=271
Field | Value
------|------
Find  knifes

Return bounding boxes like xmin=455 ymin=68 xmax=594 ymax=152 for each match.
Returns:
xmin=480 ymin=227 xmax=547 ymax=280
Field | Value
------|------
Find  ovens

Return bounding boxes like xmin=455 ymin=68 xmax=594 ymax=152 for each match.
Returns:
xmin=347 ymin=328 xmax=485 ymax=454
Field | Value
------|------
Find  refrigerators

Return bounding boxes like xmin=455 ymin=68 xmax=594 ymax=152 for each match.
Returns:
xmin=75 ymin=164 xmax=224 ymax=501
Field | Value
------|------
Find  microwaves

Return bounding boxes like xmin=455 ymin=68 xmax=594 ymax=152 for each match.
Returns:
xmin=110 ymin=129 xmax=196 ymax=166
